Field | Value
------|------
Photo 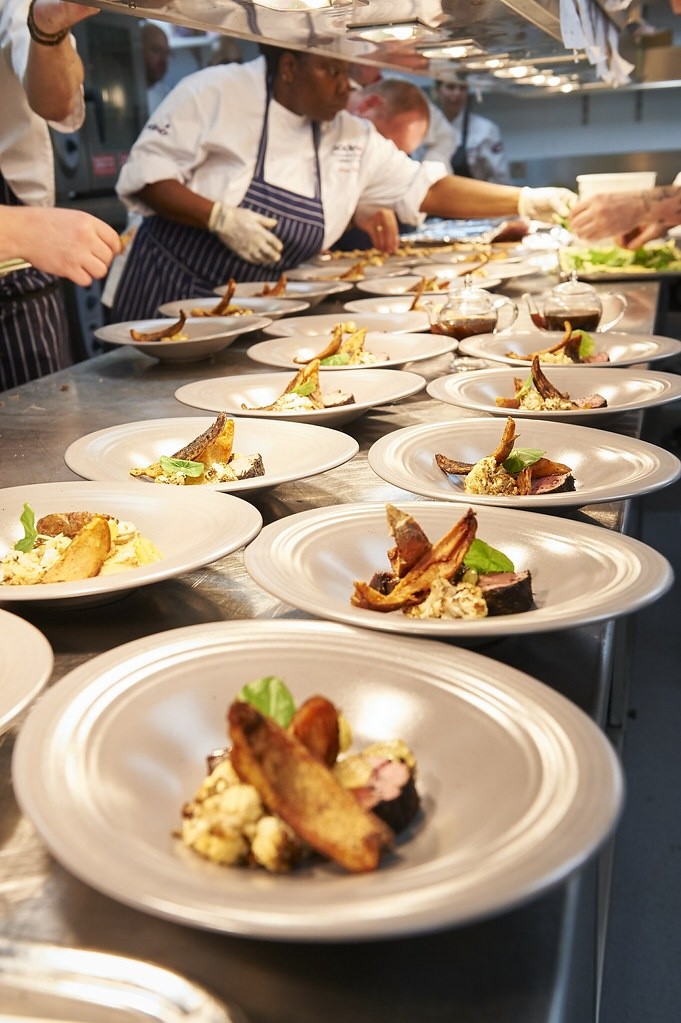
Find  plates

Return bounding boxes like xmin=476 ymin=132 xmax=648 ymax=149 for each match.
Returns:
xmin=0 ymin=482 xmax=264 ymax=607
xmin=284 ymin=256 xmax=420 ymax=282
xmin=343 ymin=294 xmax=509 ymax=313
xmin=427 ymin=367 xmax=681 ymax=416
xmin=64 ymin=418 xmax=359 ymax=492
xmin=12 ymin=618 xmax=624 ymax=941
xmin=246 ymin=333 xmax=459 ymax=369
xmin=242 ymin=502 xmax=675 ymax=634
xmin=214 ymin=282 xmax=353 ymax=307
xmin=159 ymin=298 xmax=310 ymax=321
xmin=459 ymin=332 xmax=681 ymax=366
xmin=0 ymin=609 xmax=54 ymax=736
xmin=175 ymin=370 xmax=426 ymax=427
xmin=0 ymin=936 xmax=232 ymax=1023
xmin=94 ymin=317 xmax=273 ymax=362
xmin=357 ymin=249 xmax=544 ymax=289
xmin=368 ymin=418 xmax=681 ymax=508
xmin=262 ymin=314 xmax=440 ymax=337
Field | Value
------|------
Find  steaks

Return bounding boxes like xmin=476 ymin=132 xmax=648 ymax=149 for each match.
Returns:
xmin=330 ymin=753 xmax=419 ymax=829
xmin=453 ymin=569 xmax=534 ymax=615
xmin=324 ymin=389 xmax=356 ymax=408
xmin=528 ymin=473 xmax=575 ymax=493
xmin=582 ymin=351 xmax=611 ymax=363
xmin=228 ymin=454 xmax=265 ymax=480
xmin=573 ymin=393 xmax=607 ymax=408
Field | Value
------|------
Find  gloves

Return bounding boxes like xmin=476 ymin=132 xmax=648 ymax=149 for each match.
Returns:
xmin=207 ymin=200 xmax=284 ymax=266
xmin=517 ymin=185 xmax=578 ymax=226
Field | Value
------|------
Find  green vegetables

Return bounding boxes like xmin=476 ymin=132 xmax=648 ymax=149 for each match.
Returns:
xmin=12 ymin=504 xmax=54 ymax=552
xmin=159 ymin=454 xmax=204 ymax=479
xmin=571 ymin=242 xmax=679 ymax=272
xmin=502 ymin=448 xmax=548 ymax=473
xmin=462 ymin=538 xmax=516 ymax=574
xmin=514 ymin=375 xmax=534 ymax=399
xmin=293 ymin=380 xmax=317 ymax=396
xmin=320 ymin=353 xmax=348 ymax=366
xmin=238 ymin=674 xmax=298 ymax=731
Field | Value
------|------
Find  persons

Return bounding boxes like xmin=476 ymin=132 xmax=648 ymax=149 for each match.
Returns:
xmin=562 ymin=0 xmax=681 ymax=250
xmin=0 ymin=1 xmax=125 ymax=397
xmin=340 ymin=62 xmax=430 ymax=253
xmin=346 ymin=62 xmax=464 ymax=175
xmin=430 ymin=80 xmax=512 ymax=187
xmin=140 ymin=23 xmax=173 ymax=119
xmin=99 ymin=42 xmax=578 ymax=357
xmin=204 ymin=39 xmax=243 ymax=67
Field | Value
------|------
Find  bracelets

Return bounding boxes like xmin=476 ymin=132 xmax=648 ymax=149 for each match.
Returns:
xmin=26 ymin=1 xmax=70 ymax=43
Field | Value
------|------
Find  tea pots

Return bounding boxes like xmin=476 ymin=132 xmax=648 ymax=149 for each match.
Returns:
xmin=426 ymin=273 xmax=519 ymax=342
xmin=523 ymin=271 xmax=627 ymax=332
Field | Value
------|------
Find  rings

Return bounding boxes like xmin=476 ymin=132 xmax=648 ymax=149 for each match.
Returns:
xmin=377 ymin=225 xmax=385 ymax=233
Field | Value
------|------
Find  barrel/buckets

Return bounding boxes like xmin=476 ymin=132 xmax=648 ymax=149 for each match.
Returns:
xmin=576 ymin=172 xmax=658 ymax=203
